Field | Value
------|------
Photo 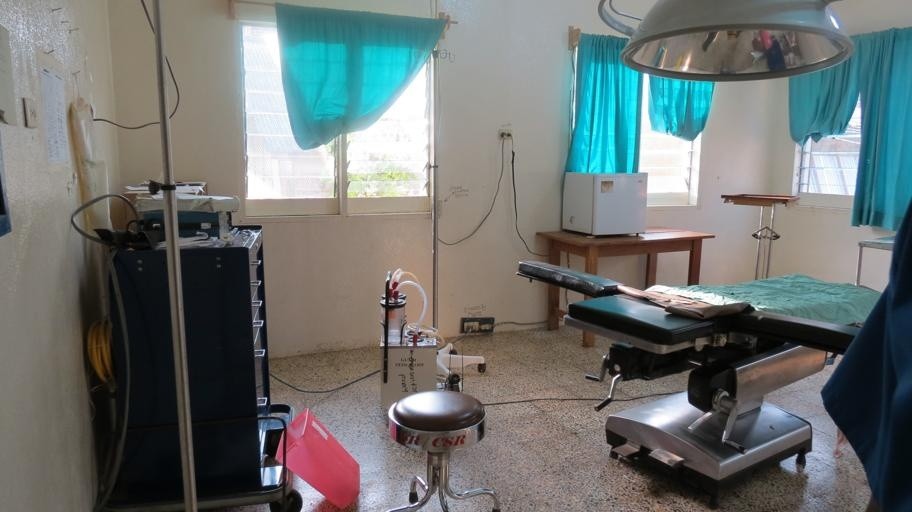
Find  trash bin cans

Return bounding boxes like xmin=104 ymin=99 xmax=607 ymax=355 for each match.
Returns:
xmin=274 ymin=407 xmax=360 ymax=510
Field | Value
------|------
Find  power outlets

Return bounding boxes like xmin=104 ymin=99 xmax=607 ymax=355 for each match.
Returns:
xmin=498 ymin=126 xmax=513 ymax=142
xmin=463 ymin=322 xmax=479 ymax=332
xmin=480 ymin=319 xmax=493 ymax=333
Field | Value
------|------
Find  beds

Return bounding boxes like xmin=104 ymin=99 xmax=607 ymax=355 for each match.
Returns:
xmin=561 ymin=270 xmax=883 ymax=509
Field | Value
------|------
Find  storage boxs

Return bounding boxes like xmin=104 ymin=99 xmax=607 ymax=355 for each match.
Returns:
xmin=121 ymin=181 xmax=214 ymax=226
xmin=137 ymin=195 xmax=240 ymax=236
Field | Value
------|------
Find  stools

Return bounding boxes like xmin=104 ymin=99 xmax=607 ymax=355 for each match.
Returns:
xmin=385 ymin=390 xmax=501 ymax=512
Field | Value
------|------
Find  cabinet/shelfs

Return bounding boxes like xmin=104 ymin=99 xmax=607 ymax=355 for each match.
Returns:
xmin=88 ymin=225 xmax=303 ymax=511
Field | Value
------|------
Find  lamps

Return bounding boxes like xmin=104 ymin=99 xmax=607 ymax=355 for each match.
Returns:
xmin=599 ymin=0 xmax=856 ymax=80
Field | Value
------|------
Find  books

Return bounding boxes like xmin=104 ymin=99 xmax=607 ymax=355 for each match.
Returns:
xmin=134 ymin=194 xmax=240 ymax=241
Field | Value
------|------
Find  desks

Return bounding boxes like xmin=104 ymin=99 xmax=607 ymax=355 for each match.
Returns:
xmin=535 ymin=229 xmax=718 ymax=347
xmin=856 ymin=237 xmax=895 ymax=285
